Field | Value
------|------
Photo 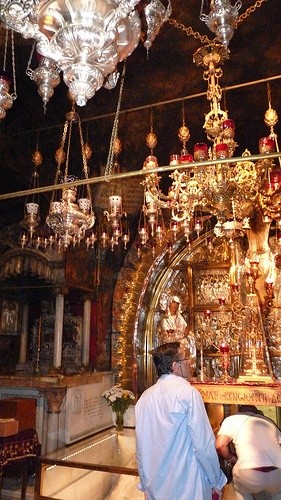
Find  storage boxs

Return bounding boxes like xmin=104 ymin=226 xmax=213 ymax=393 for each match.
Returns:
xmin=0 ymin=397 xmax=36 ymax=438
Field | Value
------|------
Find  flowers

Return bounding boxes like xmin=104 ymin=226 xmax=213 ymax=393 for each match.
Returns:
xmin=101 ymin=384 xmax=135 ymax=414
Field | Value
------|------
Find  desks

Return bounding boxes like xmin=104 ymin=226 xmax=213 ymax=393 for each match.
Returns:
xmin=0 ymin=428 xmax=39 ymax=500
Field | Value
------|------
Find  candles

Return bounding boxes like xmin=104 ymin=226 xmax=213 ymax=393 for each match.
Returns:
xmin=266 ymin=279 xmax=273 ymax=290
xmin=246 ymin=272 xmax=252 ymax=279
xmin=232 ymin=283 xmax=238 ymax=292
xmin=219 ymin=297 xmax=225 ymax=306
xmin=250 ymin=259 xmax=259 ymax=264
xmin=37 ymin=316 xmax=41 ymax=351
xmin=205 ymin=310 xmax=211 ymax=319
xmin=220 ymin=343 xmax=229 ymax=354
xmin=167 ymin=327 xmax=174 ymax=334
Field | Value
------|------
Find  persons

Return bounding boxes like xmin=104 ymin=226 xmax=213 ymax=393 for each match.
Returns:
xmin=157 ymin=294 xmax=198 ymax=376
xmin=134 ymin=342 xmax=228 ymax=500
xmin=214 ymin=405 xmax=281 ymax=500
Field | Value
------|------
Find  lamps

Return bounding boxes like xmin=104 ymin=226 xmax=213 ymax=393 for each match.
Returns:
xmin=0 ymin=0 xmax=281 ymax=261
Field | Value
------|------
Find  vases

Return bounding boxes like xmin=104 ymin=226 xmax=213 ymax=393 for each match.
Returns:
xmin=116 ymin=409 xmax=124 ymax=431
